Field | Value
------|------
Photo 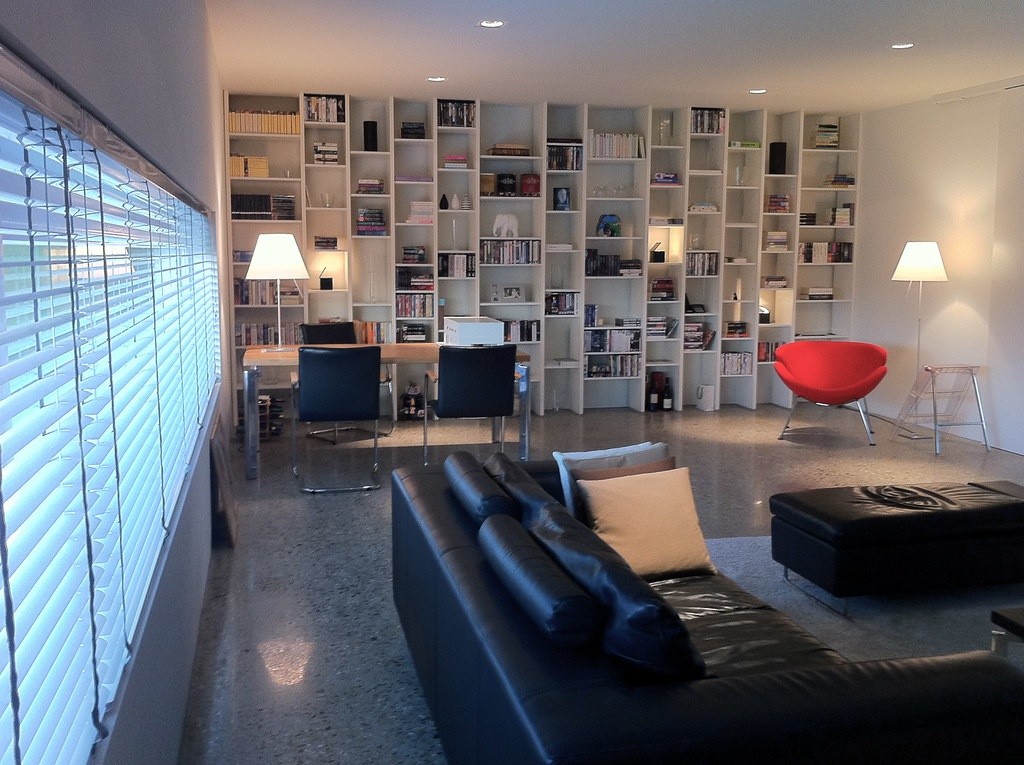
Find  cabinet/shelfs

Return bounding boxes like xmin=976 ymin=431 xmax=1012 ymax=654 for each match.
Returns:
xmin=224 ymin=87 xmax=867 ymax=434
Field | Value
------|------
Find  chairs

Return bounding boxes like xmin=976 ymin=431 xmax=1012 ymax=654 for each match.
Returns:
xmin=421 ymin=342 xmax=518 ymax=464
xmin=772 ymin=341 xmax=887 ymax=449
xmin=289 ymin=347 xmax=382 ymax=492
xmin=298 ymin=322 xmax=357 ymax=346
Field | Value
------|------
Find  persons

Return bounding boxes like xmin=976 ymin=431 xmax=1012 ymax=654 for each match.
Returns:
xmin=557 ymin=189 xmax=568 ymax=210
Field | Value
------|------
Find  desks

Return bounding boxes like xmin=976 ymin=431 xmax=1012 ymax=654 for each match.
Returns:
xmin=242 ymin=343 xmax=532 ymax=472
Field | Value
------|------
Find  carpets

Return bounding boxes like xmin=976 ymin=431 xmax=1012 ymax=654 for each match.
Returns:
xmin=702 ymin=529 xmax=1024 ymax=672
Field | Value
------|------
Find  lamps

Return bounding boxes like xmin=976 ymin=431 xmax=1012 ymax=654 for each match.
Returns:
xmin=888 ymin=238 xmax=949 ymax=439
xmin=244 ymin=234 xmax=311 ymax=352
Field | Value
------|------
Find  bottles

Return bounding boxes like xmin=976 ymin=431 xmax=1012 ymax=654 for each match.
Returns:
xmin=646 ymin=377 xmax=672 ymax=412
xmin=439 ymin=193 xmax=472 ymax=210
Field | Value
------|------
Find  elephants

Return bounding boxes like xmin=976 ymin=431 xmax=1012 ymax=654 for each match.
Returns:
xmin=493 ymin=212 xmax=519 ymax=237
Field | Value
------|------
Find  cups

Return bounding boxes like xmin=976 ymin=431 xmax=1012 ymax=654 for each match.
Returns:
xmin=735 ymin=166 xmax=744 ymax=186
xmin=595 ymin=186 xmax=607 ymax=198
xmin=551 ymin=263 xmax=563 ymax=289
xmin=657 ymin=115 xmax=671 ymax=146
xmin=616 ymin=186 xmax=627 ymax=198
xmin=320 ymin=193 xmax=335 ymax=208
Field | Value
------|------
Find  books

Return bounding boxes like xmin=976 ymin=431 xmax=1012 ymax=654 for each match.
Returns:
xmin=583 ymin=107 xmax=856 ymax=378
xmin=229 ymin=95 xmax=583 ymax=346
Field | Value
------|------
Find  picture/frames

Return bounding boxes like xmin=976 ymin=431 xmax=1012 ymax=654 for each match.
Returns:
xmin=210 ymin=411 xmax=239 ymax=548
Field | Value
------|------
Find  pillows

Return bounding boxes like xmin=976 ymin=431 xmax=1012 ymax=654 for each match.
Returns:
xmin=553 ymin=442 xmax=651 ymax=514
xmin=570 ymin=456 xmax=675 ymax=525
xmin=577 ymin=467 xmax=719 ymax=576
xmin=562 ymin=442 xmax=669 ymax=521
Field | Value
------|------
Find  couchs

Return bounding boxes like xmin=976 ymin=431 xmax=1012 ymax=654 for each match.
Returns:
xmin=770 ymin=480 xmax=1024 ymax=614
xmin=391 ymin=450 xmax=1024 ymax=765
